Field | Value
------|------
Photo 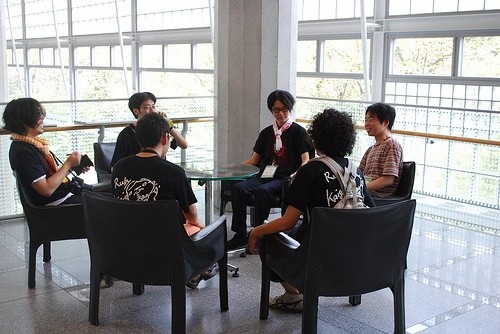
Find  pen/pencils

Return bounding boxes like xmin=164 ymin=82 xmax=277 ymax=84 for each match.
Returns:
xmin=66 ymin=154 xmax=82 ymax=156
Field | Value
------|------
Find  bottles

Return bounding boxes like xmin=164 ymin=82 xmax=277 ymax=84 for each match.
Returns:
xmin=156 ymin=110 xmax=173 ymax=128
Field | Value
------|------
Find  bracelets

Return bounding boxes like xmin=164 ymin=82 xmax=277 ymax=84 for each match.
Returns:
xmin=169 ymin=126 xmax=174 ymax=131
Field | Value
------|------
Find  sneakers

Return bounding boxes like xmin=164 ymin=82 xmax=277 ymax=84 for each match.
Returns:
xmin=268 ymin=292 xmax=304 ymax=312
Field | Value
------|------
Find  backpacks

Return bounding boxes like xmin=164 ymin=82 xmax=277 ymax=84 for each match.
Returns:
xmin=307 ymin=155 xmax=371 ymax=209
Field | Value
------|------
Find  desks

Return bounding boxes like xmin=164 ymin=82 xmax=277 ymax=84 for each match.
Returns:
xmin=173 ymin=161 xmax=260 ymax=277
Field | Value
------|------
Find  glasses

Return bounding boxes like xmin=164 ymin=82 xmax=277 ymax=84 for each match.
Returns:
xmin=140 ymin=106 xmax=158 ymax=110
xmin=272 ymin=106 xmax=288 ymax=112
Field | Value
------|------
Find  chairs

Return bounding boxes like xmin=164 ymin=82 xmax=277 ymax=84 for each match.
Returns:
xmin=13 ymin=143 xmax=415 ymax=334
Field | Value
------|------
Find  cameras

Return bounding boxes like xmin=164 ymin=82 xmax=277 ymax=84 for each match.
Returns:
xmin=72 ymin=177 xmax=94 ymax=191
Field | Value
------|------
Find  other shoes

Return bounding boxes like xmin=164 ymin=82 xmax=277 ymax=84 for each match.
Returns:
xmin=186 ymin=273 xmax=203 ymax=289
xmin=227 ymin=232 xmax=249 ymax=250
xmin=201 ymin=262 xmax=220 ymax=281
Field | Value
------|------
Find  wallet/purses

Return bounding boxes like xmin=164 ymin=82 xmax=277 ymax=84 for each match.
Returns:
xmin=72 ymin=154 xmax=93 ymax=175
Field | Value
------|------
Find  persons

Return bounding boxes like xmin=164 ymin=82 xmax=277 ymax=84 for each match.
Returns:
xmin=224 ymin=90 xmax=311 ymax=253
xmin=110 ymin=92 xmax=188 ymax=173
xmin=355 ymin=102 xmax=404 ymax=204
xmin=246 ymin=107 xmax=376 ymax=312
xmin=3 ymin=98 xmax=114 ymax=208
xmin=111 ymin=112 xmax=219 ymax=290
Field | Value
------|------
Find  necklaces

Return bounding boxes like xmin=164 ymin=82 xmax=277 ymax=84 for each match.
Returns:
xmin=139 ymin=148 xmax=160 ymax=157
